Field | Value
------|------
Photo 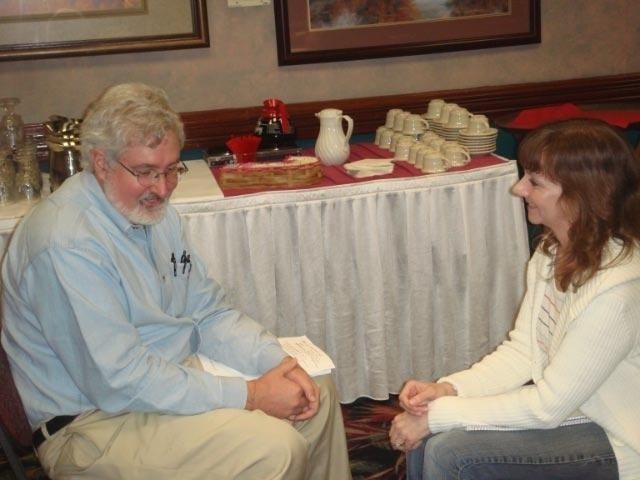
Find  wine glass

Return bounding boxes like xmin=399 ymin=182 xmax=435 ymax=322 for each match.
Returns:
xmin=0 ymin=98 xmax=43 ymax=205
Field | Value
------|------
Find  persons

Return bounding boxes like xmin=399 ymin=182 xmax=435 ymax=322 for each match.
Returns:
xmin=0 ymin=80 xmax=351 ymax=480
xmin=384 ymin=116 xmax=639 ymax=479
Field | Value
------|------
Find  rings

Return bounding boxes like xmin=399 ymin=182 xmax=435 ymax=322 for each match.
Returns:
xmin=395 ymin=438 xmax=405 ymax=445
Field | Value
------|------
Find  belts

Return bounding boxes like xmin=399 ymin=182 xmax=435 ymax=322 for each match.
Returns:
xmin=32 ymin=414 xmax=79 ymax=451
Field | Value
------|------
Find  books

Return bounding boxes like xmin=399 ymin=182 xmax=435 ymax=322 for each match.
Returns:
xmin=197 ymin=333 xmax=337 ymax=382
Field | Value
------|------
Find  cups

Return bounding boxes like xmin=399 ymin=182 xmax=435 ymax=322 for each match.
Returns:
xmin=426 ymin=99 xmax=490 ymax=135
xmin=374 ymin=108 xmax=471 ymax=174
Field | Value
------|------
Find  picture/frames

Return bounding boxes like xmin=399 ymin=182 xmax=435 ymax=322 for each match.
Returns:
xmin=273 ymin=0 xmax=542 ymax=68
xmin=1 ymin=0 xmax=212 ymax=60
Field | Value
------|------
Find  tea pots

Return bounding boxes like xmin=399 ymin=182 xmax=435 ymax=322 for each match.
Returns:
xmin=254 ymin=96 xmax=297 ymax=150
xmin=314 ymin=108 xmax=355 ymax=165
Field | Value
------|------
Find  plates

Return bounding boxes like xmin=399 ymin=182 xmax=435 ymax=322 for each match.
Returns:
xmin=422 ymin=113 xmax=499 ymax=156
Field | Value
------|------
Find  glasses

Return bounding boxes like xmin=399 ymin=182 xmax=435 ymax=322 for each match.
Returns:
xmin=116 ymin=158 xmax=190 ymax=186
